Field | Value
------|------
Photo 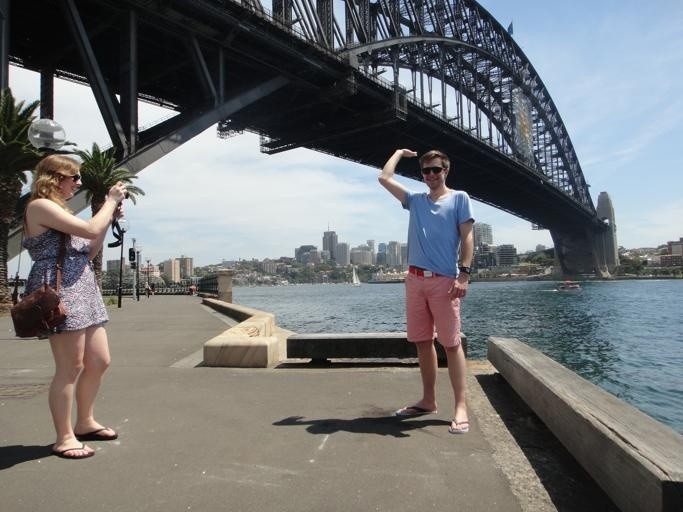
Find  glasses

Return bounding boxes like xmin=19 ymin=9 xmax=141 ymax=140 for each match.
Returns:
xmin=70 ymin=174 xmax=82 ymax=184
xmin=420 ymin=166 xmax=445 ymax=174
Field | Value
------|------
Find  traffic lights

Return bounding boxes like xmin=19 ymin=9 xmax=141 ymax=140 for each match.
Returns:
xmin=128 ymin=248 xmax=137 ymax=271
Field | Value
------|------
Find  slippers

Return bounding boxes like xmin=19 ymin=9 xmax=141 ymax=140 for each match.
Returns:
xmin=448 ymin=416 xmax=469 ymax=434
xmin=391 ymin=405 xmax=438 ymax=418
xmin=57 ymin=443 xmax=96 ymax=460
xmin=73 ymin=427 xmax=118 ymax=442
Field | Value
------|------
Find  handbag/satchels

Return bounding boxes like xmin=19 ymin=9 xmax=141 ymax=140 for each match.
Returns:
xmin=8 ymin=282 xmax=68 ymax=341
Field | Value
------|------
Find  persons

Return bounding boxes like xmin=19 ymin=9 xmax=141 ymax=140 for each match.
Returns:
xmin=376 ymin=146 xmax=475 ymax=434
xmin=19 ymin=152 xmax=127 ymax=462
xmin=149 ymin=283 xmax=155 ymax=295
xmin=188 ymin=286 xmax=193 ymax=296
xmin=143 ymin=282 xmax=151 ymax=297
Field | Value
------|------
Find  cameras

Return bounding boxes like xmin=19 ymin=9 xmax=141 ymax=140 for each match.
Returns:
xmin=105 ymin=186 xmax=129 ymax=199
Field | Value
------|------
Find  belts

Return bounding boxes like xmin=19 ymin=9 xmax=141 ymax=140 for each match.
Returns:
xmin=407 ymin=265 xmax=440 ymax=278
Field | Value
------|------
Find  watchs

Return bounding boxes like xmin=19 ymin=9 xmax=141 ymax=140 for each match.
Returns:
xmin=459 ymin=266 xmax=472 ymax=275
